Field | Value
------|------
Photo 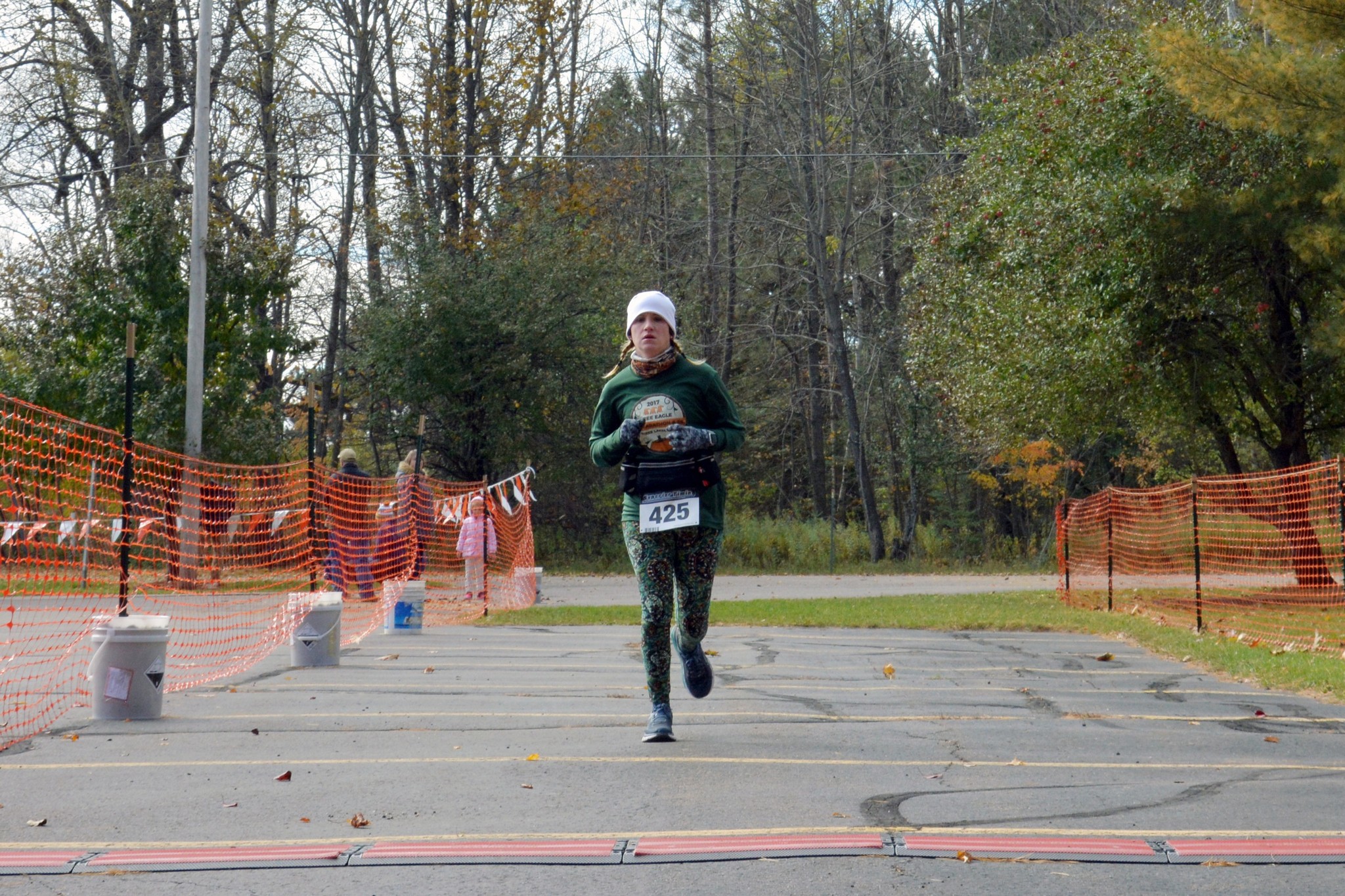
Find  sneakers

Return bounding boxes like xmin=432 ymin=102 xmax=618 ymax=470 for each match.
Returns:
xmin=672 ymin=626 xmax=714 ymax=698
xmin=642 ymin=704 xmax=677 ymax=743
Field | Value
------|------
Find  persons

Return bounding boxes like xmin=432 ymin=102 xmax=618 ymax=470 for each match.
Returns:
xmin=324 ymin=449 xmax=381 ymax=602
xmin=375 ymin=450 xmax=435 ymax=581
xmin=455 ymin=497 xmax=498 ymax=601
xmin=587 ymin=291 xmax=746 ymax=741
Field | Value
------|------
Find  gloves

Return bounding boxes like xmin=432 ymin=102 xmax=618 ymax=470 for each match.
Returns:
xmin=619 ymin=417 xmax=646 ymax=445
xmin=665 ymin=423 xmax=715 ymax=453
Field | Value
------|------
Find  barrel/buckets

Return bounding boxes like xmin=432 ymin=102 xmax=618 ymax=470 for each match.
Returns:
xmin=383 ymin=580 xmax=425 ymax=636
xmin=288 ymin=591 xmax=344 ymax=666
xmin=514 ymin=567 xmax=543 ymax=606
xmin=87 ymin=614 xmax=171 ymax=722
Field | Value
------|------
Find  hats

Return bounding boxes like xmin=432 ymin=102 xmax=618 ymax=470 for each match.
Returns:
xmin=337 ymin=448 xmax=357 ymax=461
xmin=375 ymin=500 xmax=393 ymax=522
xmin=625 ymin=291 xmax=676 ymax=343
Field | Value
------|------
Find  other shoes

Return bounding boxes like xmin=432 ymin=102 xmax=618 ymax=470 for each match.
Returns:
xmin=362 ymin=596 xmax=380 ymax=602
xmin=478 ymin=592 xmax=485 ymax=598
xmin=463 ymin=592 xmax=472 ymax=600
